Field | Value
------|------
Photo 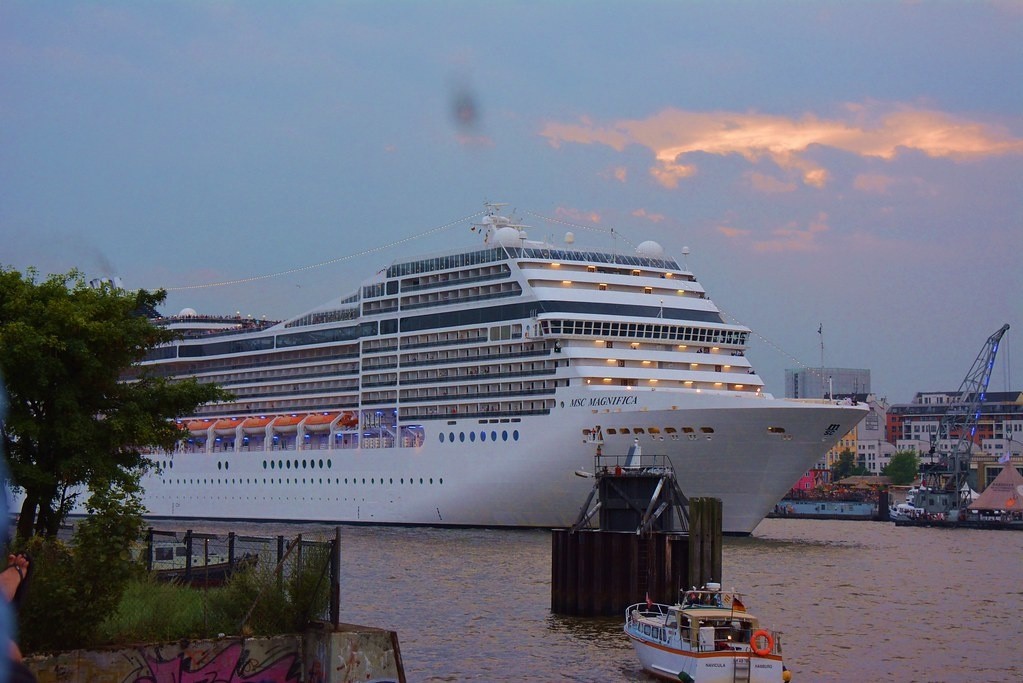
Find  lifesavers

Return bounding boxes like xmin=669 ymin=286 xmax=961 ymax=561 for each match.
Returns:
xmin=750 ymin=631 xmax=774 ymax=657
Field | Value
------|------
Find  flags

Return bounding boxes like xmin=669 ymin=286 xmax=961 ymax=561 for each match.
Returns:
xmin=733 ymin=597 xmax=746 ymax=612
xmin=646 ymin=594 xmax=653 ymax=607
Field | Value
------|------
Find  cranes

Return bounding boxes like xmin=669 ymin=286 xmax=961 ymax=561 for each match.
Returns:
xmin=888 ymin=322 xmax=1011 ymax=530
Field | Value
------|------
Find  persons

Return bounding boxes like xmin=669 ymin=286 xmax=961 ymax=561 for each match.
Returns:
xmin=714 ymin=589 xmax=722 ymax=606
xmin=156 ymin=289 xmax=371 ymax=338
xmin=681 ymin=617 xmax=751 ymax=649
xmin=412 ymin=343 xmax=534 ymax=413
xmin=697 ymin=335 xmax=755 ymax=374
xmin=0 ymin=550 xmax=37 ymax=683
xmin=904 ymin=508 xmax=948 ymax=522
xmin=959 ymin=510 xmax=1023 ymax=521
xmin=591 ymin=428 xmax=596 ymax=441
xmin=660 ymin=274 xmax=710 ymax=300
xmin=774 ymin=488 xmax=871 ymax=515
xmin=824 ymin=392 xmax=857 ymax=405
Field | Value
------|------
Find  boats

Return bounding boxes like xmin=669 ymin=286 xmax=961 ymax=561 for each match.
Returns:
xmin=624 ymin=583 xmax=792 ymax=683
xmin=130 ymin=542 xmax=259 ymax=588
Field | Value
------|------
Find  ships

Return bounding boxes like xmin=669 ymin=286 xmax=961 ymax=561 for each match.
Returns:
xmin=1 ymin=202 xmax=872 ymax=534
